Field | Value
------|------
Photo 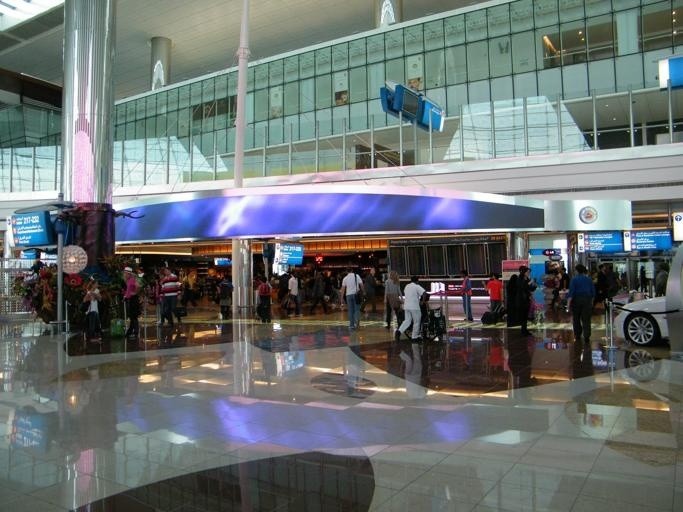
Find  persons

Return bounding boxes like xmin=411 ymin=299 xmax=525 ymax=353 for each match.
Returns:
xmin=83 ymin=259 xmax=648 ymax=343
xmin=394 ymin=342 xmax=600 ymax=416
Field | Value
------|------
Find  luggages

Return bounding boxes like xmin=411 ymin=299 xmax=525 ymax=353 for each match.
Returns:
xmin=482 ymin=304 xmax=501 ymax=325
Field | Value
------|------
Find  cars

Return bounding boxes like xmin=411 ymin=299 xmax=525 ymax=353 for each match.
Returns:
xmin=612 ymin=341 xmax=683 ymax=408
xmin=614 ymin=298 xmax=670 ymax=347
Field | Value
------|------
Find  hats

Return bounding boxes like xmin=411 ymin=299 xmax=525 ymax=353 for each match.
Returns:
xmin=123 ymin=267 xmax=133 ymax=274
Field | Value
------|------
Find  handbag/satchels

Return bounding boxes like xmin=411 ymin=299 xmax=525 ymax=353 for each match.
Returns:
xmin=354 ymin=289 xmax=364 ymax=305
xmin=175 ymin=307 xmax=186 ymax=316
